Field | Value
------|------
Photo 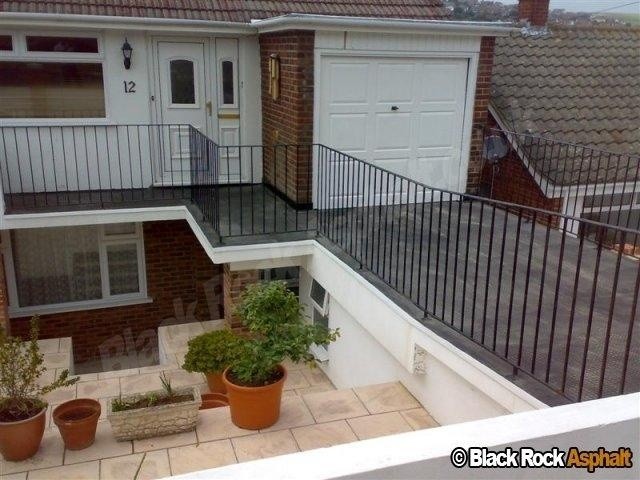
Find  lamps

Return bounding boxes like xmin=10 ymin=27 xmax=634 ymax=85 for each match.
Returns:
xmin=121 ymin=36 xmax=133 ymax=70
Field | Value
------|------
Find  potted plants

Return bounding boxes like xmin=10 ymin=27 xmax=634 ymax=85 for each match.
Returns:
xmin=0 ymin=311 xmax=79 ymax=465
xmin=106 ymin=280 xmax=342 ymax=444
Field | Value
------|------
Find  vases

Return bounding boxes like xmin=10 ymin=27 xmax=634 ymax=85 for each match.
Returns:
xmin=51 ymin=396 xmax=101 ymax=452
